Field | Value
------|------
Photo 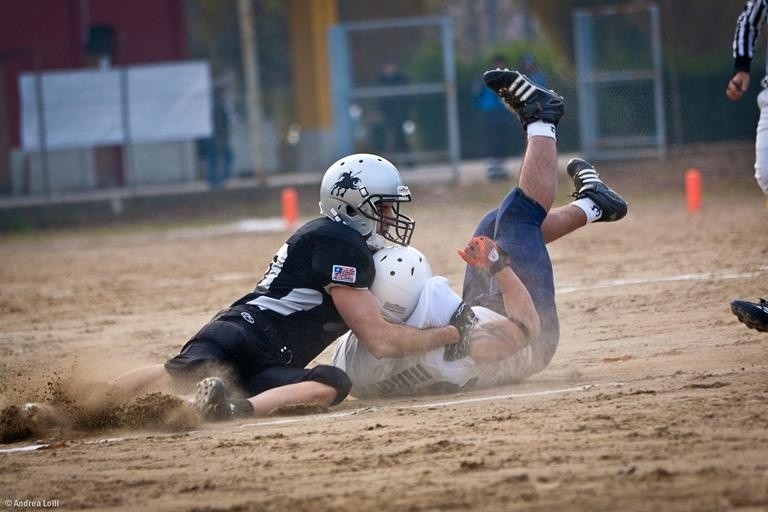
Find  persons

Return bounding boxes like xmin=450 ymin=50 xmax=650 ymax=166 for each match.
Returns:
xmin=329 ymin=69 xmax=630 ymax=402
xmin=371 ymin=60 xmax=415 ymax=167
xmin=0 ymin=153 xmax=477 ymax=435
xmin=724 ymin=2 xmax=767 ymax=331
xmin=193 ymin=60 xmax=238 ymax=190
xmin=518 ymin=53 xmax=544 ymax=85
xmin=472 ymin=52 xmax=517 ymax=178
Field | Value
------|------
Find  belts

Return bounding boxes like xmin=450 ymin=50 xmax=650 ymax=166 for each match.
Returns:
xmin=229 ymin=305 xmax=297 ymax=369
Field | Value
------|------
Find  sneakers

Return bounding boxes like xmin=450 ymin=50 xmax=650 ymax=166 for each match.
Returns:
xmin=481 ymin=67 xmax=566 ymax=130
xmin=730 ymin=297 xmax=768 ymax=333
xmin=190 ymin=377 xmax=235 ymax=423
xmin=566 ymin=157 xmax=629 ymax=224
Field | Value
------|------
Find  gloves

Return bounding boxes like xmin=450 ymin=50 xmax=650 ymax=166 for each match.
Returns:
xmin=453 ymin=311 xmax=476 ymax=343
xmin=455 ymin=235 xmax=509 ymax=279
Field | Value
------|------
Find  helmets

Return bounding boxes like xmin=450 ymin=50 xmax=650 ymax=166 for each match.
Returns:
xmin=319 ymin=151 xmax=411 ymax=236
xmin=368 ymin=244 xmax=432 ymax=325
